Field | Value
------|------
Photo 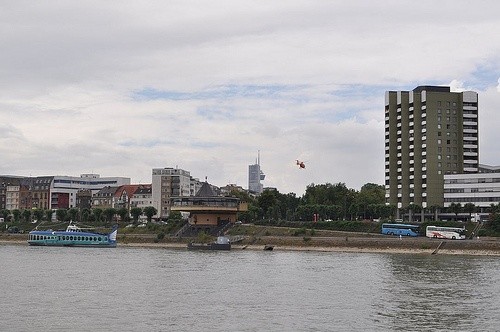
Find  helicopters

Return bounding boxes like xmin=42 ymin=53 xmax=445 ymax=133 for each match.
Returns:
xmin=296 ymin=159 xmax=306 ymax=169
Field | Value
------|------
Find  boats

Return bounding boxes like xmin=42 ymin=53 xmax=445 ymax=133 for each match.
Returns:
xmin=187 ymin=236 xmax=232 ymax=251
xmin=27 ymin=220 xmax=119 ymax=247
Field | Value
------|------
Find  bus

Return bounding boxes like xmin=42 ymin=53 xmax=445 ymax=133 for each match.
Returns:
xmin=382 ymin=223 xmax=421 ymax=237
xmin=425 ymin=225 xmax=466 ymax=240
